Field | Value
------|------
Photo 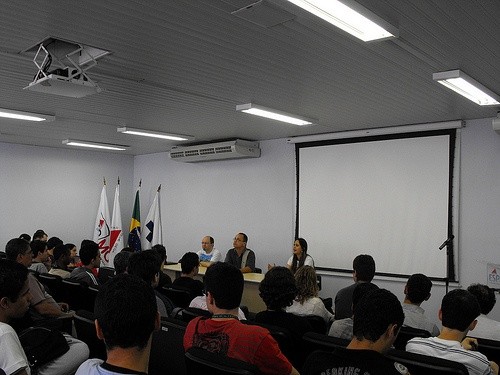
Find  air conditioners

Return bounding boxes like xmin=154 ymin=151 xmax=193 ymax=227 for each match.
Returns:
xmin=492 ymin=119 xmax=500 ymax=130
xmin=168 ymin=139 xmax=261 ymax=163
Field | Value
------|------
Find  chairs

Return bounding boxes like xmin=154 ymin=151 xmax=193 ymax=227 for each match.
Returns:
xmin=18 ymin=261 xmax=500 ymax=375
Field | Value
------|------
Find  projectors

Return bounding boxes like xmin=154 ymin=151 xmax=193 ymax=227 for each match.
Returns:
xmin=34 ymin=67 xmax=88 ymax=83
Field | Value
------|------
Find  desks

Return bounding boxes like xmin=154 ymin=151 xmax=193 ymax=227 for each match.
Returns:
xmin=163 ymin=262 xmax=268 ymax=313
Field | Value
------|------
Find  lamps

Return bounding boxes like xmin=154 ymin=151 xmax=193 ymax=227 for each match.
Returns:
xmin=433 ymin=69 xmax=500 ymax=106
xmin=235 ymin=103 xmax=318 ymax=129
xmin=61 ymin=139 xmax=131 ymax=153
xmin=287 ymin=0 xmax=400 ymax=46
xmin=116 ymin=127 xmax=195 ymax=143
xmin=0 ymin=108 xmax=56 ymax=123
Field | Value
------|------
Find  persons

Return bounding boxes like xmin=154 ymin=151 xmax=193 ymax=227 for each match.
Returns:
xmin=285 ymin=265 xmax=335 ymax=323
xmin=401 ymin=273 xmax=440 ymax=337
xmin=300 ymin=283 xmax=411 ymax=375
xmin=406 ymin=288 xmax=499 ymax=375
xmin=467 ymin=283 xmax=500 ymax=342
xmin=267 ymin=238 xmax=315 ymax=275
xmin=0 ymin=229 xmax=301 ymax=375
xmin=334 ymin=254 xmax=379 ymax=319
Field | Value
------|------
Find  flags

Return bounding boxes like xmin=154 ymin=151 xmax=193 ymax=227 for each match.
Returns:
xmin=93 ymin=185 xmax=124 ymax=275
xmin=140 ymin=192 xmax=162 ymax=251
xmin=126 ymin=190 xmax=141 ymax=251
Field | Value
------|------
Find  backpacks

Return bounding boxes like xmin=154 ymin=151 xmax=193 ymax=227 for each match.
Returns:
xmin=21 ymin=325 xmax=70 ymax=369
